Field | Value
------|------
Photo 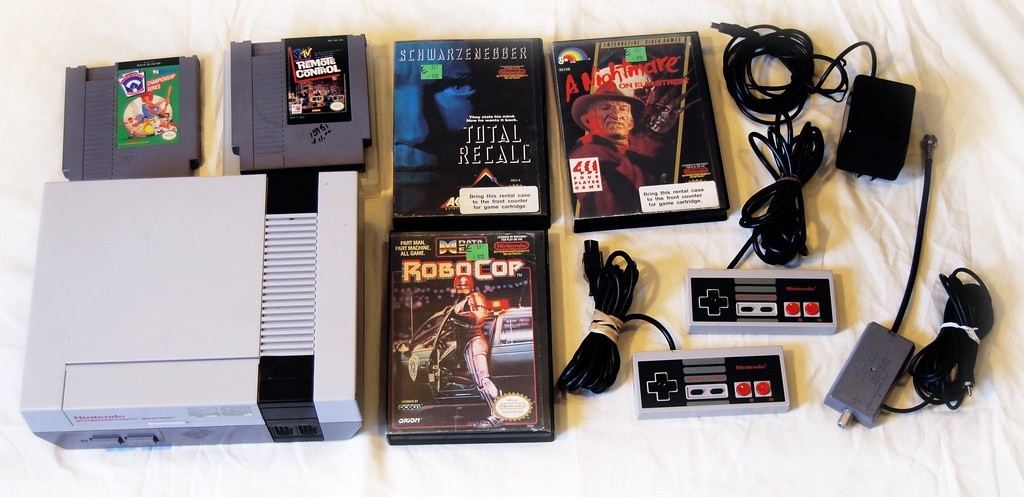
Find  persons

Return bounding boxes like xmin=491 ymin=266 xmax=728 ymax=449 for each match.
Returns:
xmin=394 ymin=41 xmax=479 ymax=214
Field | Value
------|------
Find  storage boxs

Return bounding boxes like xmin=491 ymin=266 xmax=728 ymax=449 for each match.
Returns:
xmin=549 ymin=32 xmax=731 ymax=234
xmin=390 ymin=39 xmax=552 ymax=230
xmin=386 ymin=232 xmax=555 ymax=444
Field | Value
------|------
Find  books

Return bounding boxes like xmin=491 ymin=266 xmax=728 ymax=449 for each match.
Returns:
xmin=386 ymin=232 xmax=559 ymax=443
xmin=392 ymin=37 xmax=549 ymax=229
xmin=550 ymin=30 xmax=729 ymax=232
